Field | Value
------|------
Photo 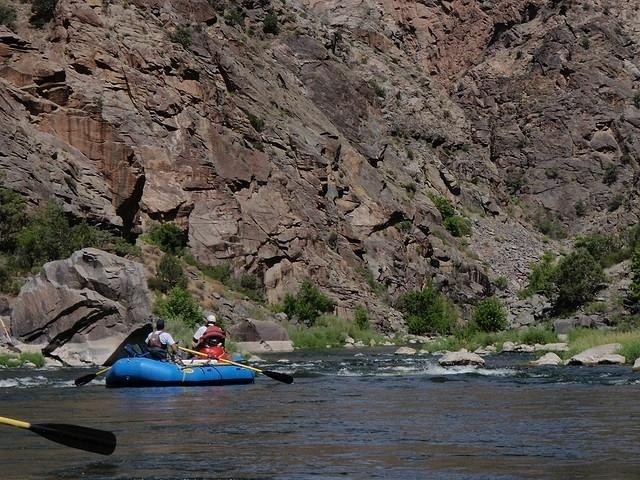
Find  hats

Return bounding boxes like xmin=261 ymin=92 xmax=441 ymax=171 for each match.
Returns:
xmin=157 ymin=320 xmax=164 ymax=329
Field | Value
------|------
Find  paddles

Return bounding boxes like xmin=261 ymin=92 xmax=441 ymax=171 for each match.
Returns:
xmin=75 ymin=341 xmax=178 ymax=387
xmin=0 ymin=416 xmax=116 ymax=455
xmin=177 ymin=346 xmax=293 ymax=384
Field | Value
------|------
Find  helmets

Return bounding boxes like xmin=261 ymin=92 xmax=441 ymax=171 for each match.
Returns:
xmin=207 ymin=315 xmax=216 ymax=323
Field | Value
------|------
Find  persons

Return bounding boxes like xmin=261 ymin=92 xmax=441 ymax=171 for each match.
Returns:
xmin=189 ymin=314 xmax=225 ymax=353
xmin=145 ymin=319 xmax=183 ymax=363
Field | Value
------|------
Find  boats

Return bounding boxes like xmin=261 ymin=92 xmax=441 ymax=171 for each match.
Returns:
xmin=105 ymin=358 xmax=255 ymax=387
